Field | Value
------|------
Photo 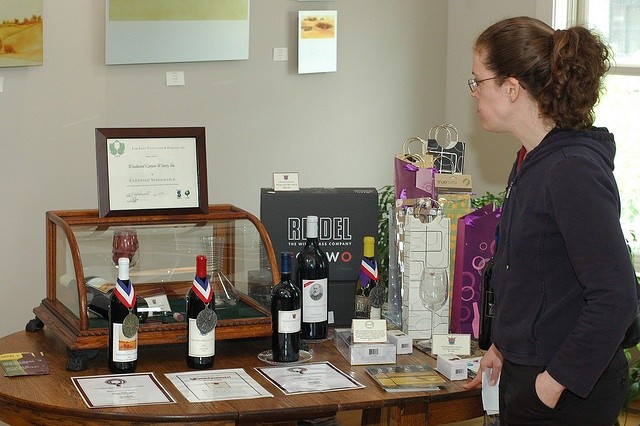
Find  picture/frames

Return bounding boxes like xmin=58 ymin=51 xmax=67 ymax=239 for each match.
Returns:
xmin=93 ymin=126 xmax=209 ymax=218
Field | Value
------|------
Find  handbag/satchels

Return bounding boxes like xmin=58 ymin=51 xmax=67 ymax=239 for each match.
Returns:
xmin=403 ymin=200 xmax=450 ymax=338
xmin=395 ymin=135 xmax=434 ymax=168
xmin=393 ymin=153 xmax=436 ymax=208
xmin=429 ymin=120 xmax=466 ymax=173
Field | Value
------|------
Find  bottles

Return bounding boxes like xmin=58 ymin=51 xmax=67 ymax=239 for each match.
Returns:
xmin=298 ymin=216 xmax=328 ymax=340
xmin=355 ymin=237 xmax=382 ymax=320
xmin=187 ymin=255 xmax=216 ymax=371
xmin=110 ymin=257 xmax=139 ymax=371
xmin=85 ymin=276 xmax=149 ymax=321
xmin=272 ymin=252 xmax=299 ymax=362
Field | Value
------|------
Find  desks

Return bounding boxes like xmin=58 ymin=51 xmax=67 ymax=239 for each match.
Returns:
xmin=0 ymin=326 xmax=490 ymax=426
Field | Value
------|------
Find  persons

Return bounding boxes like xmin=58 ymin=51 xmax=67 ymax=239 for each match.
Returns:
xmin=468 ymin=16 xmax=637 ymax=426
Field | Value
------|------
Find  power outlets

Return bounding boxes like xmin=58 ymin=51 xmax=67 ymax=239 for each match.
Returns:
xmin=165 ymin=71 xmax=185 ymax=86
xmin=273 ymin=48 xmax=288 ymax=61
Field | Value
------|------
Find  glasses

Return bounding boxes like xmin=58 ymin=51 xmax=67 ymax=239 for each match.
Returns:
xmin=468 ymin=74 xmax=526 ymax=91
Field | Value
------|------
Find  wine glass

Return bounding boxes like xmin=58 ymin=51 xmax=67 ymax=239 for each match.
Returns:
xmin=113 ymin=228 xmax=140 ymax=270
xmin=419 ymin=267 xmax=448 ymax=350
xmin=418 ymin=197 xmax=442 ymax=228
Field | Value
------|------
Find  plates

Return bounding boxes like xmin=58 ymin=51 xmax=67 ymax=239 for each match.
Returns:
xmin=256 ymin=348 xmax=313 ymax=367
xmin=298 ymin=330 xmax=335 ymax=344
